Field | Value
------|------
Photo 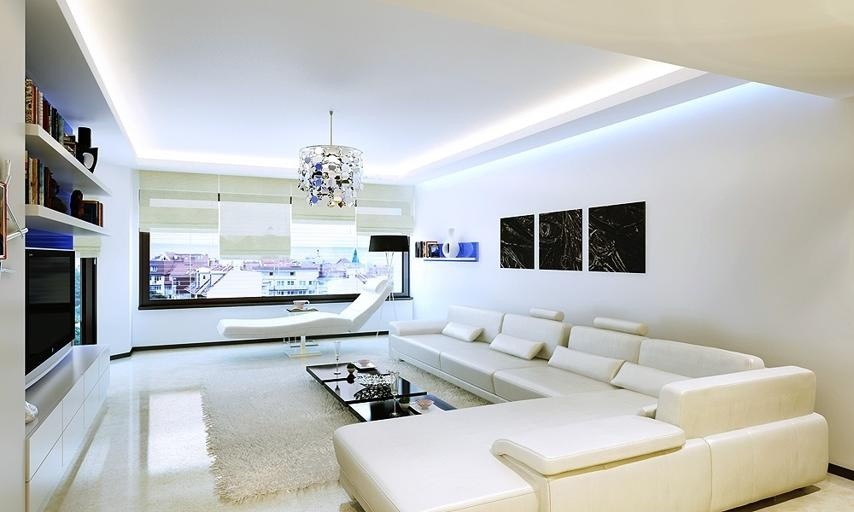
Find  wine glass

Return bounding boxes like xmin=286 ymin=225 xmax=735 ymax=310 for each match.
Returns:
xmin=333 ymin=342 xmax=342 ymax=374
xmin=389 ymin=371 xmax=399 ymax=416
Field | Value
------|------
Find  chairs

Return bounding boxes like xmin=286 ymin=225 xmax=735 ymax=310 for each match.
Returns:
xmin=216 ymin=277 xmax=394 ymax=360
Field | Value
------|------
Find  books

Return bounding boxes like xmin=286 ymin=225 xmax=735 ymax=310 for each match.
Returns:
xmin=25 ymin=77 xmax=104 ymax=227
xmin=415 ymin=241 xmax=437 ymax=257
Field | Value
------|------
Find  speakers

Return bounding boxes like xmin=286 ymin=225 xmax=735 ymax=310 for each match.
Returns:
xmin=80 ymin=257 xmax=97 ymax=345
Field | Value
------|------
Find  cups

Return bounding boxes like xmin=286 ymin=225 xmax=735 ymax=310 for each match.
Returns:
xmin=293 ymin=300 xmax=310 ymax=310
xmin=399 ymin=396 xmax=410 ymax=412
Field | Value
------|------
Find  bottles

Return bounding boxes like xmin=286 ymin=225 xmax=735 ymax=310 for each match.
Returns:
xmin=346 ymin=363 xmax=355 ymax=373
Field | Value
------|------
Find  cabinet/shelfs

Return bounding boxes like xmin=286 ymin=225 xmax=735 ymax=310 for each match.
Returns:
xmin=25 ymin=345 xmax=112 ymax=512
xmin=25 ymin=123 xmax=116 ymax=238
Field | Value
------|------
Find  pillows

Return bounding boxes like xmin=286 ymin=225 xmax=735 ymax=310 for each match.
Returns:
xmin=441 ymin=307 xmax=695 ymax=420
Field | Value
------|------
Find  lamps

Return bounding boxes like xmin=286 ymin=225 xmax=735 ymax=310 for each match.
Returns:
xmin=295 ymin=109 xmax=365 ymax=210
xmin=368 ymin=234 xmax=409 ymax=339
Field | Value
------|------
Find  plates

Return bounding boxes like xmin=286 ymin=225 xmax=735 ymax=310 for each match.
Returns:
xmin=351 ymin=362 xmax=377 ymax=369
xmin=286 ymin=307 xmax=318 ymax=312
xmin=409 ymin=402 xmax=445 ymax=414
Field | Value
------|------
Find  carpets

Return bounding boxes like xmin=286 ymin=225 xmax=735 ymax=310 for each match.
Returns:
xmin=199 ymin=361 xmax=493 ymax=507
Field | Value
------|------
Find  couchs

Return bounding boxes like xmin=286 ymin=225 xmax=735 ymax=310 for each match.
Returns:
xmin=333 ymin=304 xmax=830 ymax=511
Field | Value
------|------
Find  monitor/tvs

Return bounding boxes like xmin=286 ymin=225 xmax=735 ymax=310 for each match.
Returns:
xmin=25 ymin=247 xmax=75 ymax=391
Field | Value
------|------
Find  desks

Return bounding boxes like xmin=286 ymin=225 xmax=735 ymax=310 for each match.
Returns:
xmin=285 ymin=307 xmax=319 ymax=348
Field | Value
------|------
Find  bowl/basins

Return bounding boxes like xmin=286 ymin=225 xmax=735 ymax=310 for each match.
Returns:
xmin=416 ymin=399 xmax=433 ymax=408
xmin=359 ymin=359 xmax=370 ymax=366
